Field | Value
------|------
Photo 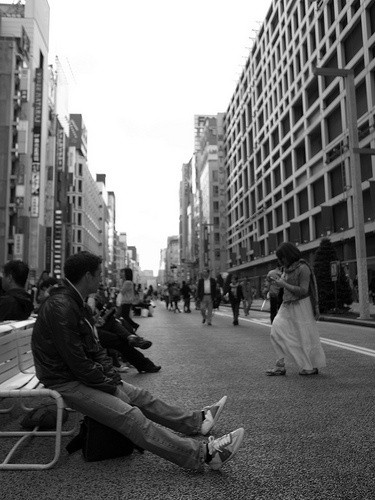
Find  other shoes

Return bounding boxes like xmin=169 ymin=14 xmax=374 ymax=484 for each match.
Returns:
xmin=202 ymin=320 xmax=205 ymax=323
xmin=233 ymin=321 xmax=238 ymax=325
xmin=114 ymin=366 xmax=129 ymax=372
xmin=127 ymin=336 xmax=152 ymax=349
xmin=140 ymin=365 xmax=161 ymax=373
xmin=265 ymin=367 xmax=286 ymax=376
xmin=207 ymin=323 xmax=212 ymax=326
xmin=299 ymin=368 xmax=318 ymax=375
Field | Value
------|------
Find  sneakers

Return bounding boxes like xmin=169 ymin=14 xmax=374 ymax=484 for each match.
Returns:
xmin=204 ymin=427 xmax=244 ymax=471
xmin=201 ymin=395 xmax=227 ymax=435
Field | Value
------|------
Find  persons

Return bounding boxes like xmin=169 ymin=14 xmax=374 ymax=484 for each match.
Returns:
xmin=0 ymin=242 xmax=327 ymax=376
xmin=31 ymin=250 xmax=245 ymax=474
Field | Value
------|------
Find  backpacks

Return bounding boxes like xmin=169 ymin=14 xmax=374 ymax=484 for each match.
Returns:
xmin=65 ymin=413 xmax=145 ymax=463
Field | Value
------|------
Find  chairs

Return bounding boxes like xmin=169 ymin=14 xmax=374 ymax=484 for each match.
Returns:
xmin=0 ymin=316 xmax=79 ymax=469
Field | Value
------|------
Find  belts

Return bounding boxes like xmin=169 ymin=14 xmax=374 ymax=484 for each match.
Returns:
xmin=204 ymin=293 xmax=211 ymax=295
